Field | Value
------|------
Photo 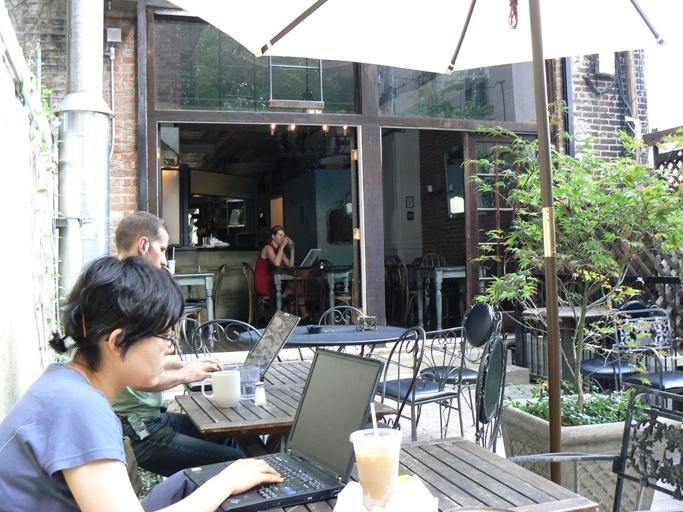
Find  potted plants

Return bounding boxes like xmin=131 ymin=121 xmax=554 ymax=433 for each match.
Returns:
xmin=458 ymin=100 xmax=683 ymax=512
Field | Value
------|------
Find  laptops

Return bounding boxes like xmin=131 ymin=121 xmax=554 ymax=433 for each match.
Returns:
xmin=280 ymin=248 xmax=322 ymax=268
xmin=184 ymin=348 xmax=384 ymax=512
xmin=187 ymin=310 xmax=302 ymax=390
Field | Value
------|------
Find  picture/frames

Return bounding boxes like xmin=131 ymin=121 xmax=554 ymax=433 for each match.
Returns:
xmin=406 ymin=197 xmax=414 ymax=208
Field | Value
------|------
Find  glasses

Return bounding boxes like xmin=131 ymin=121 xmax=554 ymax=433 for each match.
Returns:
xmin=151 ymin=329 xmax=175 ymax=352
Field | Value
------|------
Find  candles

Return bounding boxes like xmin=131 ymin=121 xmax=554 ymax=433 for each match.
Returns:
xmin=427 ymin=185 xmax=433 ymax=192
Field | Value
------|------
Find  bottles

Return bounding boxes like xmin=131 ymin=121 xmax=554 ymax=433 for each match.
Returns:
xmin=254 ymin=382 xmax=267 ymax=405
xmin=319 ymin=261 xmax=324 ymax=269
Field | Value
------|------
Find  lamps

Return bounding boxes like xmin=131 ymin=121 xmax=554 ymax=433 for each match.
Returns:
xmin=448 ymin=190 xmax=464 ymax=214
xmin=266 ymin=125 xmax=350 ymax=170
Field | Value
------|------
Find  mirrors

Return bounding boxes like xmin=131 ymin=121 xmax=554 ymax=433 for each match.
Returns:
xmin=443 ymin=142 xmax=465 ymax=219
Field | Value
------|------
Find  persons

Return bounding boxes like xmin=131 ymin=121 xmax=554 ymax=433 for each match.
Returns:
xmin=0 ymin=256 xmax=285 ymax=512
xmin=254 ymin=225 xmax=317 ymax=324
xmin=112 ymin=211 xmax=245 ymax=478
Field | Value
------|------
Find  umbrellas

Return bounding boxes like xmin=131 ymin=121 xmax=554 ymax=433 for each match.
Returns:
xmin=166 ymin=1 xmax=683 ymax=486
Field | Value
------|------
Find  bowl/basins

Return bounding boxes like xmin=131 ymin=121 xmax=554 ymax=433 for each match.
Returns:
xmin=307 ymin=326 xmax=323 ymax=333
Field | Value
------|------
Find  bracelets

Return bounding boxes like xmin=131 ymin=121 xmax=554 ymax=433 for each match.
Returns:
xmin=278 ymin=247 xmax=284 ymax=249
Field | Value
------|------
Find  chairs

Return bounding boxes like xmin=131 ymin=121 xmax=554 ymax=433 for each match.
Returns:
xmin=184 ymin=263 xmax=227 ymax=340
xmin=363 ymin=299 xmax=507 ymax=454
xmin=318 ymin=305 xmax=377 ymax=359
xmin=580 ymin=299 xmax=651 ymax=397
xmin=384 ymin=252 xmax=451 ymax=326
xmin=315 ymin=259 xmax=332 ymax=267
xmin=500 ymin=389 xmax=683 ymax=512
xmin=613 ymin=307 xmax=683 ymax=411
xmin=240 ymin=261 xmax=285 ymax=331
xmin=191 ymin=319 xmax=282 ymax=361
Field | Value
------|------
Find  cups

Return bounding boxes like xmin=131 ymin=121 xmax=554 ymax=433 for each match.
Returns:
xmin=168 ymin=260 xmax=176 ymax=274
xmin=201 ymin=369 xmax=241 ymax=408
xmin=348 ymin=428 xmax=413 ymax=507
